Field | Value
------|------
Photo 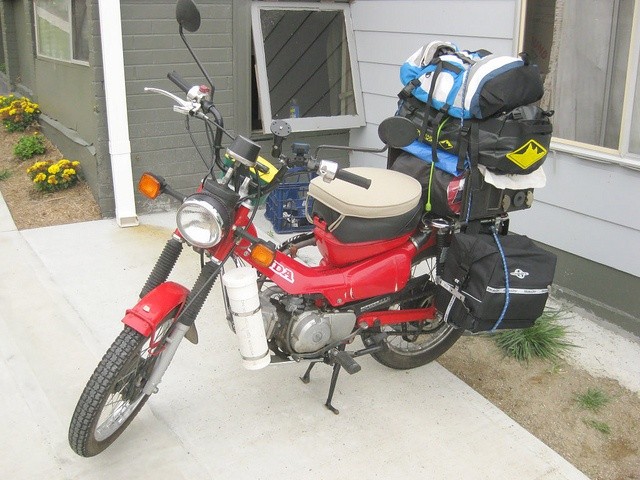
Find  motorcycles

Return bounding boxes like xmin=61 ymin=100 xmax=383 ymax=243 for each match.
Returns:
xmin=67 ymin=1 xmax=509 ymax=460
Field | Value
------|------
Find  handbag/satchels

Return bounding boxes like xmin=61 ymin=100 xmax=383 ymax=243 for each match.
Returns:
xmin=395 ymin=92 xmax=552 ymax=175
xmin=433 ymin=221 xmax=557 ymax=334
xmin=398 ymin=39 xmax=544 ymax=120
xmin=389 ymin=152 xmax=467 ymax=217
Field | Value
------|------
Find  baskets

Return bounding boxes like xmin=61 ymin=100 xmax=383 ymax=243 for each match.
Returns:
xmin=265 ymin=166 xmax=318 ymax=233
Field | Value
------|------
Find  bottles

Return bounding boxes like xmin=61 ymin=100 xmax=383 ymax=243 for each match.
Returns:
xmin=290 ymin=97 xmax=301 ymax=119
xmin=300 ymin=200 xmax=310 ymax=216
